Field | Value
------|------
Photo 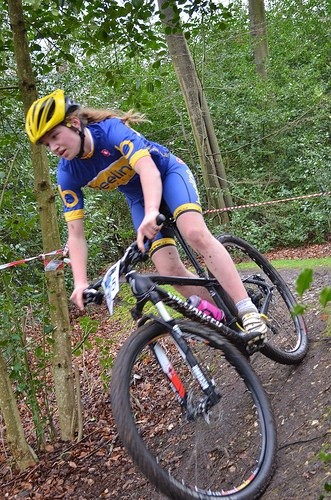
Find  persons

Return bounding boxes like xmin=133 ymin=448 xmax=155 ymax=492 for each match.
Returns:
xmin=24 ymin=89 xmax=268 ymax=344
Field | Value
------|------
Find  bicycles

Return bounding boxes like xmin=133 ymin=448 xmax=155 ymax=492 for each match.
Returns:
xmin=82 ymin=214 xmax=306 ymax=500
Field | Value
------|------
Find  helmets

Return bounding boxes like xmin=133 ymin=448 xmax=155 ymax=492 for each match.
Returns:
xmin=25 ymin=89 xmax=80 ymax=145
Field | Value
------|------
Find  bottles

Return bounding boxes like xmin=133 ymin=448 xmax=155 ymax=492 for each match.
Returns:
xmin=185 ymin=294 xmax=225 ymax=322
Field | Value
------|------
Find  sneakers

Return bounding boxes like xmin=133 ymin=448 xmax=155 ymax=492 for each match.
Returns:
xmin=242 ymin=312 xmax=267 ymax=342
